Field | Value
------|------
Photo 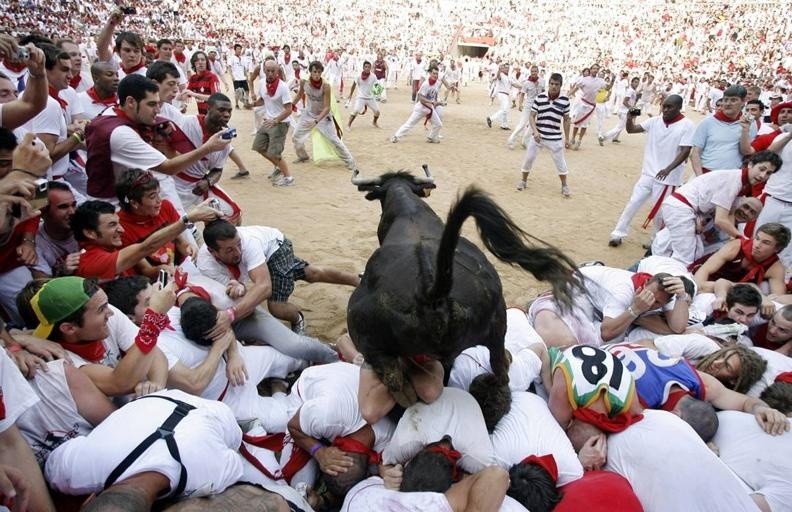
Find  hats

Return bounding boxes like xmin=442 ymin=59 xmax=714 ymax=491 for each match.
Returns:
xmin=769 ymin=95 xmax=783 ymax=102
xmin=29 ymin=276 xmax=91 ymax=340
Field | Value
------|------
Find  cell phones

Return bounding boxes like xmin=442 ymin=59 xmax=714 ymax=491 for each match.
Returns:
xmin=159 ymin=269 xmax=166 ymax=290
xmin=124 ymin=7 xmax=136 ymax=14
xmin=630 ymin=109 xmax=640 ymax=116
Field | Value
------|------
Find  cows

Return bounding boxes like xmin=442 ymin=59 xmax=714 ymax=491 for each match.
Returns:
xmin=346 ymin=164 xmax=598 ymax=435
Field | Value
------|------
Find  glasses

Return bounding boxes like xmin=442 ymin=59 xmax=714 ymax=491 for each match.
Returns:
xmin=132 ymin=170 xmax=154 ymax=192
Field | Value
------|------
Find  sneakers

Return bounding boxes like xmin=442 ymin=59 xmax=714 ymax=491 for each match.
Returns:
xmin=562 ymin=185 xmax=571 ymax=196
xmin=612 ymin=140 xmax=621 ymax=143
xmin=231 ymin=171 xmax=249 ymax=179
xmin=392 ymin=135 xmax=400 ymax=143
xmin=599 ymin=135 xmax=604 ymax=146
xmin=517 ymin=180 xmax=527 ymax=190
xmin=487 ymin=117 xmax=493 ymax=128
xmin=291 ymin=310 xmax=304 ymax=334
xmin=427 ymin=137 xmax=440 ymax=143
xmin=609 ymin=238 xmax=621 ymax=248
xmin=293 ymin=156 xmax=309 ymax=163
xmin=273 ymin=166 xmax=280 ymax=178
xmin=272 ymin=176 xmax=296 ymax=186
xmin=571 ymin=138 xmax=581 ymax=150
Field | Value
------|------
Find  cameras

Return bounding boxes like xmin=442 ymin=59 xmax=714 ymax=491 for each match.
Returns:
xmin=211 ymin=199 xmax=220 ymax=208
xmin=17 ymin=179 xmax=49 ymax=220
xmin=9 ymin=47 xmax=31 ymax=62
xmin=222 ymin=127 xmax=237 ymax=139
xmin=743 ymin=112 xmax=754 ymax=122
